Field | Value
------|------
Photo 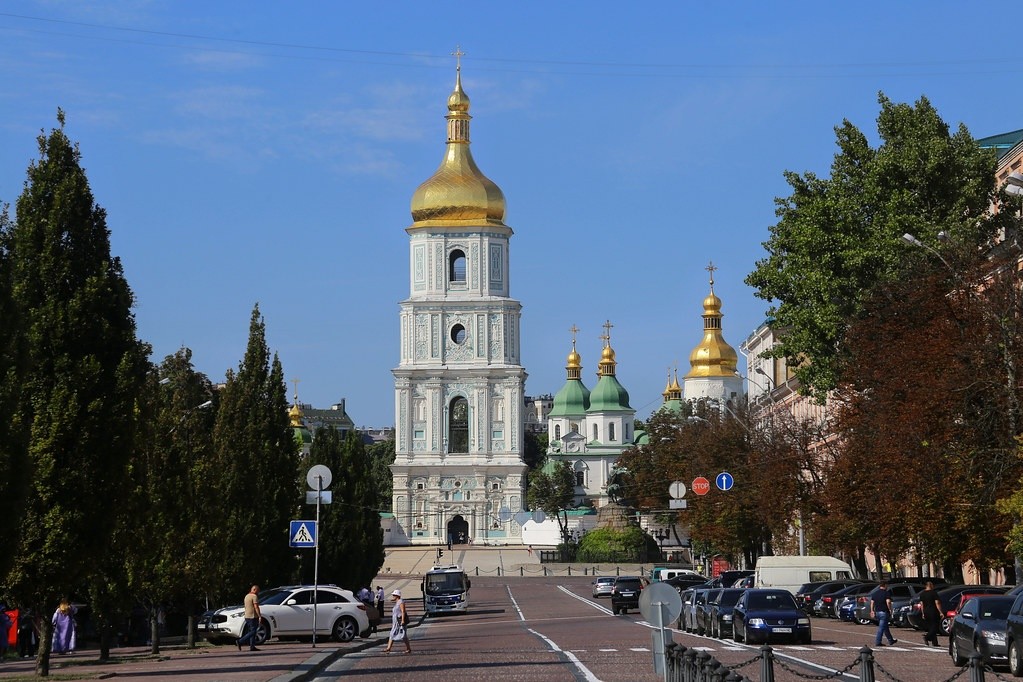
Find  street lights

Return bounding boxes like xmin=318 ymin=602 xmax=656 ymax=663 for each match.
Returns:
xmin=655 ymin=529 xmax=670 ymax=560
xmin=904 ymin=233 xmax=1022 ymax=584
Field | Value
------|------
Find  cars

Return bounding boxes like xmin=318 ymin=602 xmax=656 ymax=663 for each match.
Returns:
xmin=948 ymin=582 xmax=1023 ymax=677
xmin=661 ymin=575 xmax=709 ymax=593
xmin=688 ymin=578 xmax=718 ymax=590
xmin=678 ymin=590 xmax=719 ymax=636
xmin=591 ymin=577 xmax=615 ymax=598
xmin=709 ymin=588 xmax=746 ymax=639
xmin=731 ymin=575 xmax=755 ymax=589
xmin=796 ymin=576 xmax=1014 ymax=635
xmin=732 ymin=589 xmax=811 ymax=644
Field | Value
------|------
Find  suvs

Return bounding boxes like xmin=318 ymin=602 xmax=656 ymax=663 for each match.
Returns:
xmin=718 ymin=569 xmax=754 ymax=589
xmin=611 ymin=576 xmax=651 ymax=615
xmin=197 ymin=584 xmax=370 ymax=645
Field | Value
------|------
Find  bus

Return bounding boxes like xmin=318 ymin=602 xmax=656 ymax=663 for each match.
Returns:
xmin=421 ymin=564 xmax=471 ymax=616
xmin=649 ymin=567 xmax=697 ymax=582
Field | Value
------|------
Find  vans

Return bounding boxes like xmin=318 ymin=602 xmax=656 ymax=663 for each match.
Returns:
xmin=659 ymin=570 xmax=706 ymax=580
xmin=754 ymin=556 xmax=854 ymax=596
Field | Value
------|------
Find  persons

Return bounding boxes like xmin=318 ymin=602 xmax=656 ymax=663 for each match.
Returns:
xmin=356 ymin=586 xmax=383 ymax=619
xmin=236 ymin=585 xmax=262 ymax=651
xmin=0 ymin=597 xmax=79 ymax=660
xmin=870 ymin=583 xmax=898 ymax=647
xmin=528 ymin=545 xmax=533 ymax=557
xmin=381 ymin=589 xmax=411 ymax=653
xmin=921 ymin=582 xmax=944 ymax=647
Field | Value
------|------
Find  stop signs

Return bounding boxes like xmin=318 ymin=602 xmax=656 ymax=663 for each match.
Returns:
xmin=692 ymin=477 xmax=710 ymax=495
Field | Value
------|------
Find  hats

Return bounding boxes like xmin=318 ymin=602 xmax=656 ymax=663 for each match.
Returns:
xmin=390 ymin=590 xmax=402 ymax=596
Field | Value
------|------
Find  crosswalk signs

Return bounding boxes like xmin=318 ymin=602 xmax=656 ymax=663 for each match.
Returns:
xmin=290 ymin=521 xmax=316 ymax=548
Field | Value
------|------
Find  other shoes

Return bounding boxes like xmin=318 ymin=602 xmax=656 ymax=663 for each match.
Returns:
xmin=932 ymin=644 xmax=941 ymax=646
xmin=236 ymin=640 xmax=242 ymax=650
xmin=20 ymin=657 xmax=28 ymax=660
xmin=876 ymin=643 xmax=886 ymax=646
xmin=380 ymin=649 xmax=390 ymax=654
xmin=27 ymin=656 xmax=36 ymax=659
xmin=403 ymin=649 xmax=411 ymax=653
xmin=250 ymin=647 xmax=261 ymax=650
xmin=891 ymin=639 xmax=897 ymax=645
xmin=923 ymin=637 xmax=929 ymax=646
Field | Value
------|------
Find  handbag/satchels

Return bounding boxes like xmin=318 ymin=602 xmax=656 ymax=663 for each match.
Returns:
xmin=31 ymin=632 xmax=36 ymax=644
xmin=397 ymin=613 xmax=410 ymax=626
